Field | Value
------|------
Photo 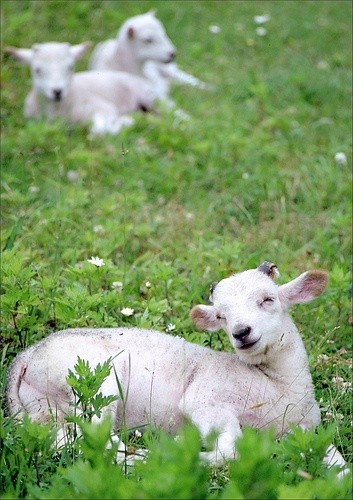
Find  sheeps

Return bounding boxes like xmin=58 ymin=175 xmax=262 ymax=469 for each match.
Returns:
xmin=5 ymin=262 xmax=349 ymax=484
xmin=4 ymin=40 xmax=159 ymax=141
xmin=89 ymin=9 xmax=215 ymax=133
